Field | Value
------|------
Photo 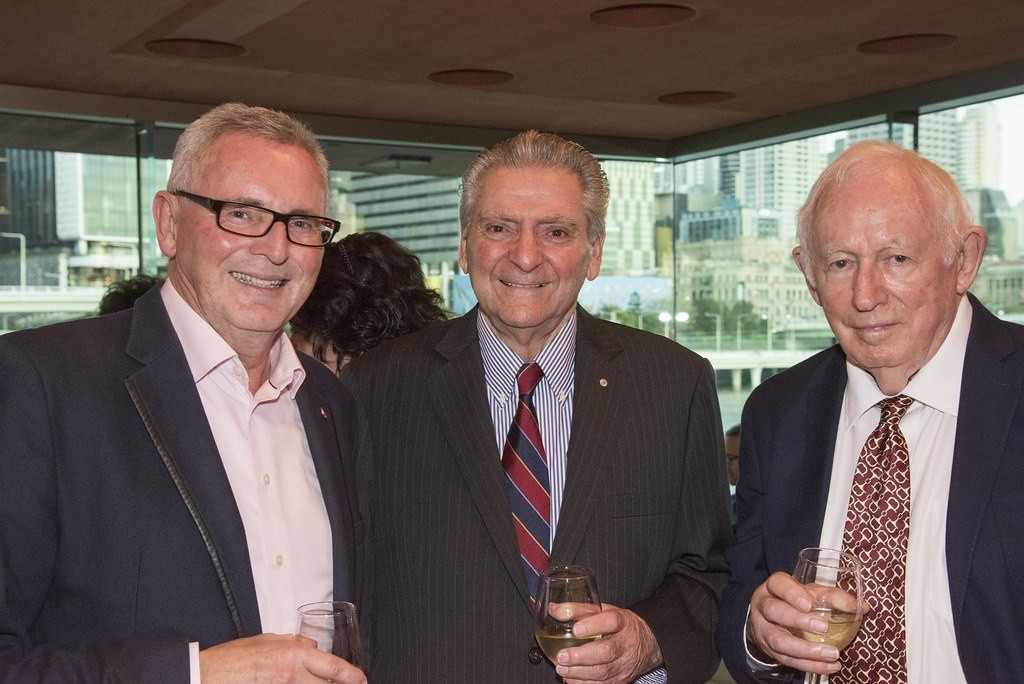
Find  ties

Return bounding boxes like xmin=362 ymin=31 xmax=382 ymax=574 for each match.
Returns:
xmin=828 ymin=398 xmax=917 ymax=684
xmin=502 ymin=363 xmax=550 ymax=610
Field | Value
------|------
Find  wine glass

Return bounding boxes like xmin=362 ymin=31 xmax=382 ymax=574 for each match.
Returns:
xmin=783 ymin=548 xmax=864 ymax=684
xmin=533 ymin=566 xmax=603 ymax=684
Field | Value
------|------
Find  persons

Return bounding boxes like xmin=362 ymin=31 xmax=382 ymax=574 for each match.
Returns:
xmin=337 ymin=128 xmax=731 ymax=683
xmin=286 ymin=231 xmax=455 ymax=379
xmin=725 ymin=425 xmax=742 ymax=526
xmin=0 ymin=102 xmax=371 ymax=684
xmin=713 ymin=140 xmax=1023 ymax=684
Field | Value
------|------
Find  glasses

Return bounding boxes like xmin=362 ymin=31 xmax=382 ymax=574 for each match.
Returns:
xmin=173 ymin=189 xmax=342 ymax=246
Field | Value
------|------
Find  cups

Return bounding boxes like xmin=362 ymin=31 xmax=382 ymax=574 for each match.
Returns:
xmin=296 ymin=600 xmax=366 ymax=683
